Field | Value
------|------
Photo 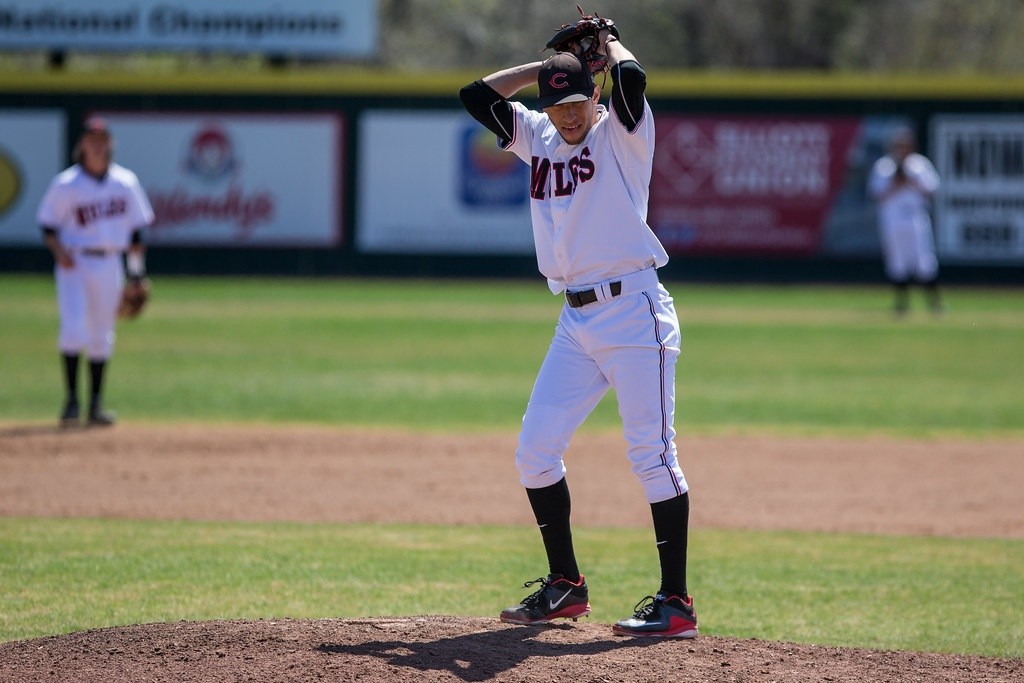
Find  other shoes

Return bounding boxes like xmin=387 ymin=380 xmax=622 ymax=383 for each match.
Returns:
xmin=89 ymin=411 xmax=113 ymax=425
xmin=61 ymin=400 xmax=81 ymax=420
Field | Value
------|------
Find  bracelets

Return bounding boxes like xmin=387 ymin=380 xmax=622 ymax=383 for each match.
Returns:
xmin=542 ymin=61 xmax=544 ymax=65
xmin=605 ymin=38 xmax=617 ymax=56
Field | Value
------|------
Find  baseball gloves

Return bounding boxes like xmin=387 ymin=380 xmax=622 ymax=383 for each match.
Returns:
xmin=118 ymin=280 xmax=149 ymax=320
xmin=546 ymin=17 xmax=621 ymax=77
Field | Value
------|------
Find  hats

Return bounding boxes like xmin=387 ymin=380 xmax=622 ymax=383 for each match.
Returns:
xmin=81 ymin=118 xmax=108 ymax=134
xmin=536 ymin=52 xmax=595 ymax=108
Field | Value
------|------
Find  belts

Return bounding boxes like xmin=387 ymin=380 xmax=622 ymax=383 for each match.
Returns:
xmin=83 ymin=248 xmax=104 ymax=257
xmin=565 ymin=281 xmax=621 ymax=308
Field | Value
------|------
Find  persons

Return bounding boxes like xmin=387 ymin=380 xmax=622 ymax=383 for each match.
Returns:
xmin=459 ymin=4 xmax=699 ymax=638
xmin=37 ymin=126 xmax=154 ymax=424
xmin=868 ymin=129 xmax=945 ymax=312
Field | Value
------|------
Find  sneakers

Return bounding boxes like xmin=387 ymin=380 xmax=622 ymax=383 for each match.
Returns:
xmin=613 ymin=591 xmax=699 ymax=638
xmin=499 ymin=573 xmax=591 ymax=625
